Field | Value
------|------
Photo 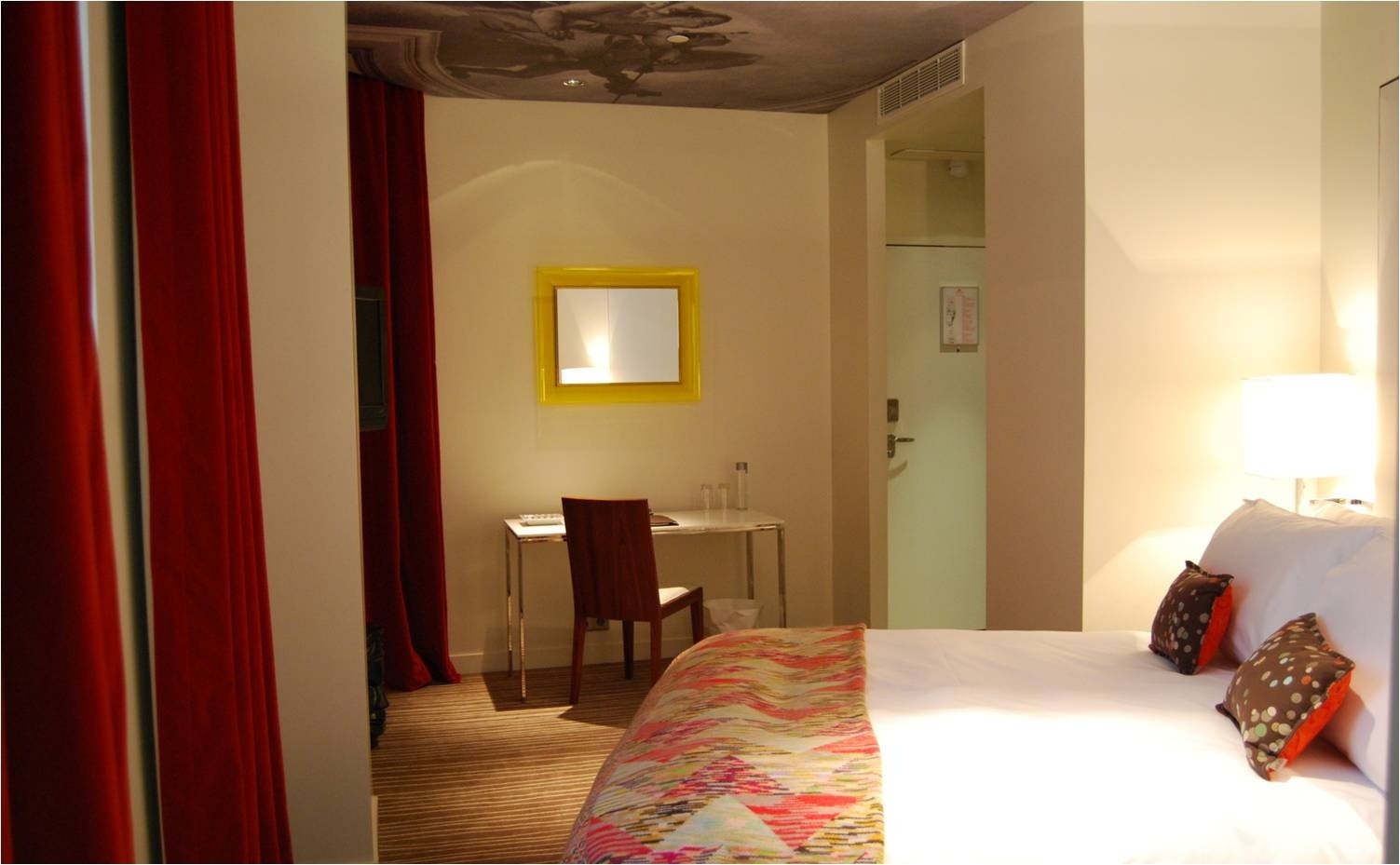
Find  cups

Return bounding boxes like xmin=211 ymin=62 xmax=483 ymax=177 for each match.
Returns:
xmin=718 ymin=484 xmax=730 ymax=509
xmin=699 ymin=484 xmax=714 ymax=511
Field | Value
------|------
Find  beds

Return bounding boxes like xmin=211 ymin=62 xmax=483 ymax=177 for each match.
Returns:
xmin=557 ymin=631 xmax=1396 ymax=864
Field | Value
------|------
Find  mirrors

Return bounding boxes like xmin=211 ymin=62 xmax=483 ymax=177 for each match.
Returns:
xmin=532 ymin=265 xmax=701 ymax=405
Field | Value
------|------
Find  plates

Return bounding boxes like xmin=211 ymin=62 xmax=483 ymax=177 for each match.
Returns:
xmin=520 ymin=514 xmax=565 ymax=526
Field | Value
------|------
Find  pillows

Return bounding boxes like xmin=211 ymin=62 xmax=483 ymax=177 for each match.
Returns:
xmin=1197 ymin=498 xmax=1395 ymax=792
xmin=1145 ymin=554 xmax=1239 ymax=674
xmin=1215 ymin=612 xmax=1359 ymax=783
xmin=1307 ymin=499 xmax=1394 ymax=526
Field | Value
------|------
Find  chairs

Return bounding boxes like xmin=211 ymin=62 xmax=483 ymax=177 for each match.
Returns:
xmin=561 ymin=496 xmax=705 ymax=703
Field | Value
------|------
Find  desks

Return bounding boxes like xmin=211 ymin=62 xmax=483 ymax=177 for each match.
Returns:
xmin=501 ymin=510 xmax=788 ymax=701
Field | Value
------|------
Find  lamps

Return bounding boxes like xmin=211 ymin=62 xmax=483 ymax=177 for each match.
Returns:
xmin=1241 ymin=374 xmax=1367 ymax=512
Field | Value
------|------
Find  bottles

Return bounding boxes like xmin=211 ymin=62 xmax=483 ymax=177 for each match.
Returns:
xmin=736 ymin=462 xmax=749 ymax=510
